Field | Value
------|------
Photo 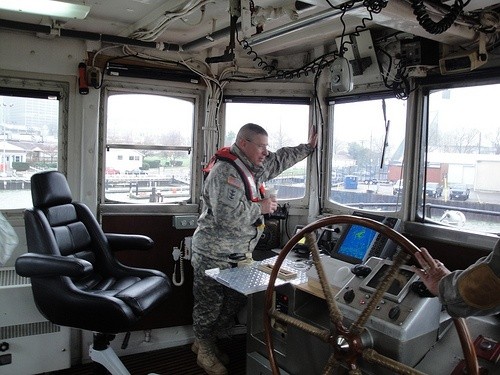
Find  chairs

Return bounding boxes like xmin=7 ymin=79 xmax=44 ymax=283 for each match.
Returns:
xmin=15 ymin=170 xmax=172 ymax=375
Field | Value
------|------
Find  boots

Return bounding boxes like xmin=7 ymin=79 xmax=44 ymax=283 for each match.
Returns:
xmin=192 ymin=336 xmax=228 ymax=365
xmin=196 ymin=339 xmax=227 ymax=375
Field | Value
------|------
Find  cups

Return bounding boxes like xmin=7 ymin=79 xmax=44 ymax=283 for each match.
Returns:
xmin=264 ymin=189 xmax=278 ymax=202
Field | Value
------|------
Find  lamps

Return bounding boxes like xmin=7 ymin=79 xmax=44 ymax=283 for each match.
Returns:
xmin=439 ymin=210 xmax=466 ymax=228
xmin=0 ymin=0 xmax=90 ymax=20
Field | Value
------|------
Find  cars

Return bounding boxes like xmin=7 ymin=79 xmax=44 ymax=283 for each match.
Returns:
xmin=422 ymin=182 xmax=443 ymax=198
xmin=449 ymin=185 xmax=471 ymax=200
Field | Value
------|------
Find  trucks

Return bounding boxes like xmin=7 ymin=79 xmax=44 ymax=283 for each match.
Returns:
xmin=362 ymin=178 xmax=378 ymax=184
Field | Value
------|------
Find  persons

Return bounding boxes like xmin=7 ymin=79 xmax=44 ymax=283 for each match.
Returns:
xmin=414 ymin=240 xmax=500 ymax=319
xmin=191 ymin=123 xmax=318 ymax=375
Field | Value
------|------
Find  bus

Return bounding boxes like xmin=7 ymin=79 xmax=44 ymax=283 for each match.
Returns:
xmin=344 ymin=175 xmax=358 ymax=189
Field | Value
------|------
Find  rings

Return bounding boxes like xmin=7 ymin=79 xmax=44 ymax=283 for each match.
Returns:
xmin=437 ymin=263 xmax=444 ymax=267
xmin=424 ymin=266 xmax=431 ymax=271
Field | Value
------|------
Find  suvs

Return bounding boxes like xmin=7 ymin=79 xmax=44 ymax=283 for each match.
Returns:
xmin=393 ymin=179 xmax=403 ymax=195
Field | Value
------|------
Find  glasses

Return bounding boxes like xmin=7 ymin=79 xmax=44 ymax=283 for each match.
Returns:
xmin=245 ymin=141 xmax=270 ymax=150
